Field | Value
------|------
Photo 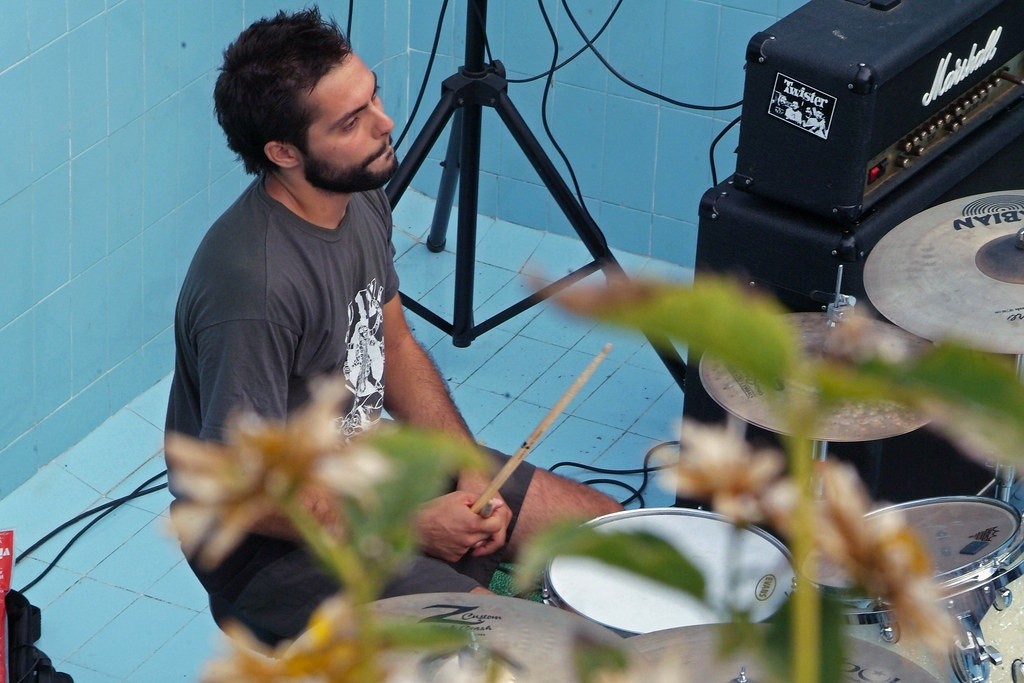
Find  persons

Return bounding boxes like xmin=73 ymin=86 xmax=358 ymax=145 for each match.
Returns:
xmin=167 ymin=4 xmax=624 ymax=656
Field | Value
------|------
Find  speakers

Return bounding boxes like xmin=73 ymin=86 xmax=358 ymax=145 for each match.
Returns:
xmin=677 ymin=98 xmax=1024 ymax=538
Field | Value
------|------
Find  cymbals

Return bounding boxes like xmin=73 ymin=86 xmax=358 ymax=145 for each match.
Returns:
xmin=694 ymin=310 xmax=941 ymax=444
xmin=269 ymin=590 xmax=942 ymax=683
xmin=860 ymin=186 xmax=1024 ymax=356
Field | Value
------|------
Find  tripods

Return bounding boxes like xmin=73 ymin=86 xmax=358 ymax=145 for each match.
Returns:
xmin=385 ymin=0 xmax=688 ymax=395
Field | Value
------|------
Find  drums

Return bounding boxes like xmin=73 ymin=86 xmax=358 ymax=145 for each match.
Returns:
xmin=801 ymin=493 xmax=1024 ymax=683
xmin=540 ymin=504 xmax=799 ymax=638
